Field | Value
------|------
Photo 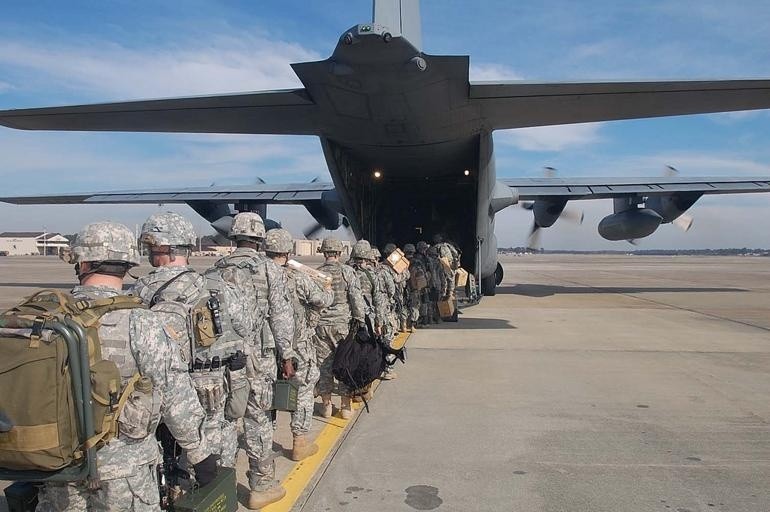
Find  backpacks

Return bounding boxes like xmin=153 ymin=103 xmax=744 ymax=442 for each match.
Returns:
xmin=0 ymin=288 xmax=151 ymax=483
xmin=147 ymin=299 xmax=196 ymax=366
xmin=331 ymin=311 xmax=387 ymax=390
xmin=216 ymin=262 xmax=260 ymax=328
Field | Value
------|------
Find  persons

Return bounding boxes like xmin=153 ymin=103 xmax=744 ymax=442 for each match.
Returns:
xmin=33 ymin=219 xmax=217 ymax=511
xmin=119 ymin=211 xmax=251 ymax=512
xmin=213 ymin=211 xmax=293 ymax=506
xmin=308 ymin=236 xmax=363 ymax=418
xmin=258 ymin=228 xmax=335 ymax=461
xmin=344 ymin=239 xmax=462 ymax=347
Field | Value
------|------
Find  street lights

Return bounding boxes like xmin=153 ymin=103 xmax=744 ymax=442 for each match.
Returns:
xmin=42 ymin=224 xmax=48 ymax=256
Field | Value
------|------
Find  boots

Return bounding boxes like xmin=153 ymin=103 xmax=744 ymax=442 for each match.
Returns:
xmin=291 ymin=432 xmax=320 ymax=462
xmin=318 ymin=392 xmax=355 ymax=419
xmin=401 ymin=319 xmax=417 ymax=334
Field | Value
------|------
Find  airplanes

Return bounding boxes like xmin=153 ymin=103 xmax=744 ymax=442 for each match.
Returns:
xmin=0 ymin=0 xmax=770 ymax=296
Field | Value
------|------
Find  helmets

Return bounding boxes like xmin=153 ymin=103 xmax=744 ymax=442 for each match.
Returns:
xmin=384 ymin=243 xmax=397 ymax=254
xmin=227 ymin=211 xmax=267 ymax=245
xmin=138 ymin=210 xmax=198 ymax=268
xmin=318 ymin=237 xmax=345 ymax=258
xmin=349 ymin=239 xmax=382 ymax=262
xmin=403 ymin=240 xmax=429 ymax=255
xmin=70 ymin=221 xmax=142 ymax=280
xmin=260 ymin=228 xmax=294 ymax=257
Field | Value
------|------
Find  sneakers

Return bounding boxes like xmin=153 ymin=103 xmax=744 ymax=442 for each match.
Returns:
xmin=383 ymin=370 xmax=398 ymax=380
xmin=247 ymin=486 xmax=287 ymax=510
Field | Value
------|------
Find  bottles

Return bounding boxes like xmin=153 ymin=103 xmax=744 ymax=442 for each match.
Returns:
xmin=132 ymin=374 xmax=156 ymax=437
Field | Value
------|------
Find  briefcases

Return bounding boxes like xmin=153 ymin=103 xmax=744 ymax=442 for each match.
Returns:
xmin=172 ymin=465 xmax=238 ymax=512
xmin=271 ymin=379 xmax=300 ymax=413
